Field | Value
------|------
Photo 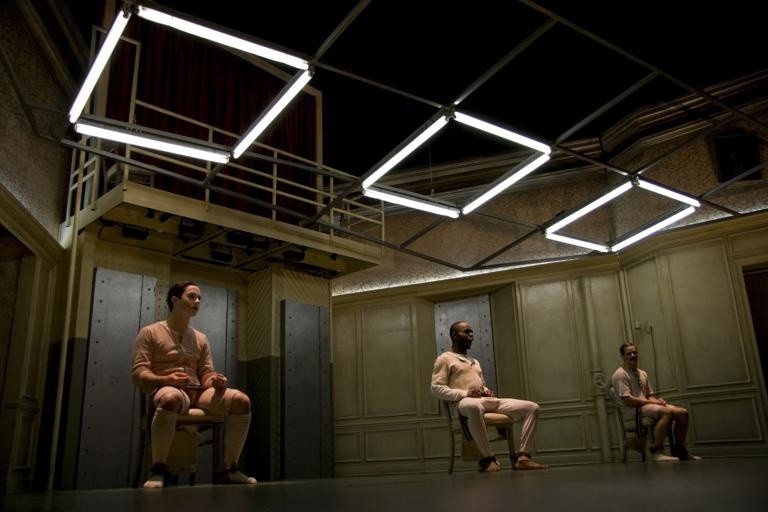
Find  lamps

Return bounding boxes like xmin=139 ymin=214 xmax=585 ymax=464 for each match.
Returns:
xmin=65 ymin=5 xmax=314 ymax=167
xmin=359 ymin=104 xmax=553 ymax=218
xmin=541 ymin=173 xmax=702 ymax=252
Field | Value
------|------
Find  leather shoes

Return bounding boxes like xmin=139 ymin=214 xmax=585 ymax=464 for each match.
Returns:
xmin=478 ymin=455 xmax=502 ymax=472
xmin=513 ymin=459 xmax=549 ymax=469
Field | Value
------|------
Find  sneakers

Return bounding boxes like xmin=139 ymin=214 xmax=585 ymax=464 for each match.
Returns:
xmin=651 ymin=448 xmax=680 ymax=462
xmin=219 ymin=467 xmax=257 ymax=484
xmin=671 ymin=446 xmax=703 ymax=460
xmin=143 ymin=471 xmax=165 ymax=488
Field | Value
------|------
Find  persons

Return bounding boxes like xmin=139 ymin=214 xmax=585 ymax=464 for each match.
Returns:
xmin=131 ymin=281 xmax=259 ymax=488
xmin=430 ymin=322 xmax=550 ymax=472
xmin=612 ymin=343 xmax=704 ymax=461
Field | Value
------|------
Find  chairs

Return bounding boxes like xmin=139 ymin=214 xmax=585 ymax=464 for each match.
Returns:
xmin=133 ymin=381 xmax=228 ymax=487
xmin=608 ymin=383 xmax=677 ymax=465
xmin=441 ymin=395 xmax=517 ymax=475
